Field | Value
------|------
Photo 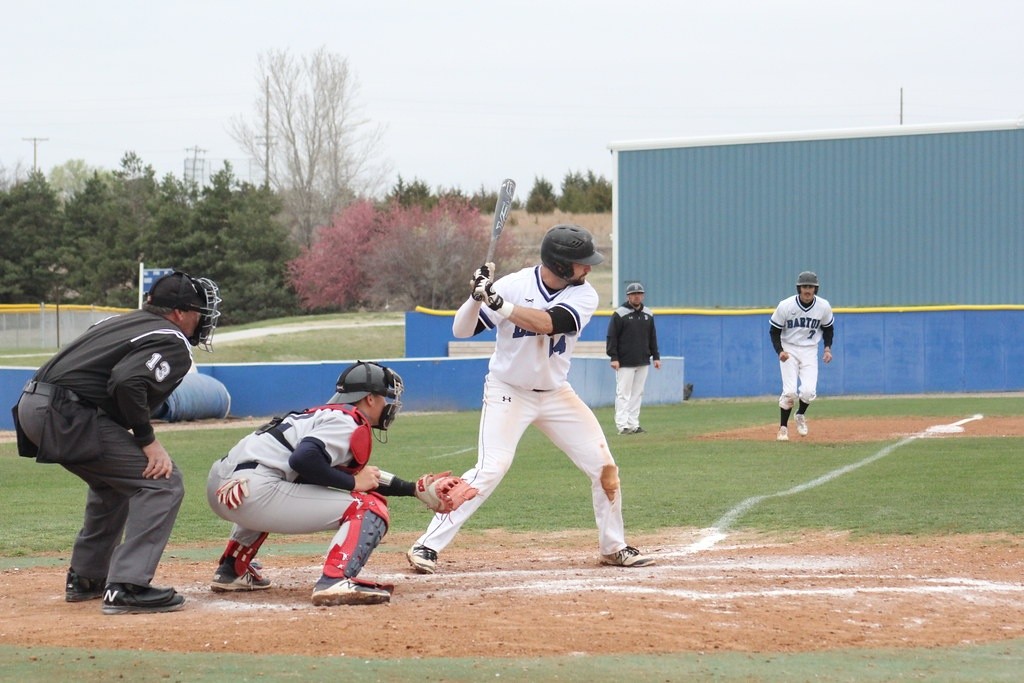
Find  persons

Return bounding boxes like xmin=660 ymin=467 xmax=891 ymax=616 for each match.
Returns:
xmin=768 ymin=272 xmax=835 ymax=441
xmin=206 ymin=353 xmax=478 ymax=604
xmin=408 ymin=223 xmax=656 ymax=574
xmin=606 ymin=282 xmax=661 ymax=435
xmin=11 ymin=270 xmax=222 ymax=612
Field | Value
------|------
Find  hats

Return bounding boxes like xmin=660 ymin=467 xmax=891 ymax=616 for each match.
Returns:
xmin=149 ymin=272 xmax=205 ymax=308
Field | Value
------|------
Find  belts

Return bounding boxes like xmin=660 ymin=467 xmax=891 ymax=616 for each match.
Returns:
xmin=25 ymin=380 xmax=80 ymax=403
xmin=220 ymin=454 xmax=260 ymax=472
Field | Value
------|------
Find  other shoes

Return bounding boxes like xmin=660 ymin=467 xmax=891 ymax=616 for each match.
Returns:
xmin=619 ymin=426 xmax=647 ymax=435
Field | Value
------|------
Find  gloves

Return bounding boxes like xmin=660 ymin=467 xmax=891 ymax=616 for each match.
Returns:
xmin=470 ymin=263 xmax=497 ymax=290
xmin=473 ymin=277 xmax=503 ymax=311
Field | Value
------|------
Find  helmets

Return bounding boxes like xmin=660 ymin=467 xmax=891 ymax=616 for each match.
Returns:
xmin=626 ymin=283 xmax=645 ymax=297
xmin=795 ymin=272 xmax=819 ymax=295
xmin=540 ymin=225 xmax=604 ymax=280
xmin=326 ymin=362 xmax=389 ymax=404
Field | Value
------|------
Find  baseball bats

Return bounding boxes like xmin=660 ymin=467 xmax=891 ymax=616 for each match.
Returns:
xmin=473 ymin=178 xmax=516 ymax=301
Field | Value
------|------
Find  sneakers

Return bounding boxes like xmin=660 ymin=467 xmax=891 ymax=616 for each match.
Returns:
xmin=210 ymin=562 xmax=272 ymax=595
xmin=310 ymin=573 xmax=390 ymax=606
xmin=793 ymin=410 xmax=808 ymax=436
xmin=66 ymin=569 xmax=108 ymax=602
xmin=777 ymin=426 xmax=789 ymax=441
xmin=102 ymin=577 xmax=186 ymax=613
xmin=406 ymin=541 xmax=438 ymax=575
xmin=601 ymin=543 xmax=656 ymax=568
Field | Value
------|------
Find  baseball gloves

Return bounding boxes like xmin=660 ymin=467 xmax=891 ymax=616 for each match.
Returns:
xmin=416 ymin=472 xmax=477 ymax=513
